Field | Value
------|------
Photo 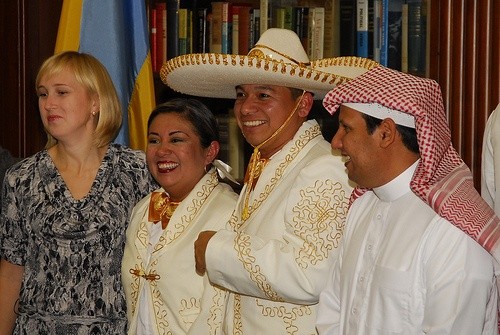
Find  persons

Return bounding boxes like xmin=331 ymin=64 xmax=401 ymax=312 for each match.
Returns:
xmin=481 ymin=105 xmax=500 ymax=218
xmin=0 ymin=50 xmax=159 ymax=335
xmin=315 ymin=65 xmax=500 ymax=335
xmin=161 ymin=28 xmax=380 ymax=335
xmin=121 ymin=98 xmax=241 ymax=335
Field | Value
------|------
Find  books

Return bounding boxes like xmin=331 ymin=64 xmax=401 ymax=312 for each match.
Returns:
xmin=147 ymin=0 xmax=426 ymax=190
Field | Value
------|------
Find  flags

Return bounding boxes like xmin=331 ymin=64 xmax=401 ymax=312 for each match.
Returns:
xmin=44 ymin=0 xmax=155 ymax=151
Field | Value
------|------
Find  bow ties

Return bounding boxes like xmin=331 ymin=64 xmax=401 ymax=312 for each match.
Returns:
xmin=243 ymin=153 xmax=271 ymax=191
xmin=147 ymin=191 xmax=181 ymax=230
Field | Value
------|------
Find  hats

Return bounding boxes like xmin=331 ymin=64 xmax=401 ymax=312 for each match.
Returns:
xmin=159 ymin=27 xmax=381 ymax=99
